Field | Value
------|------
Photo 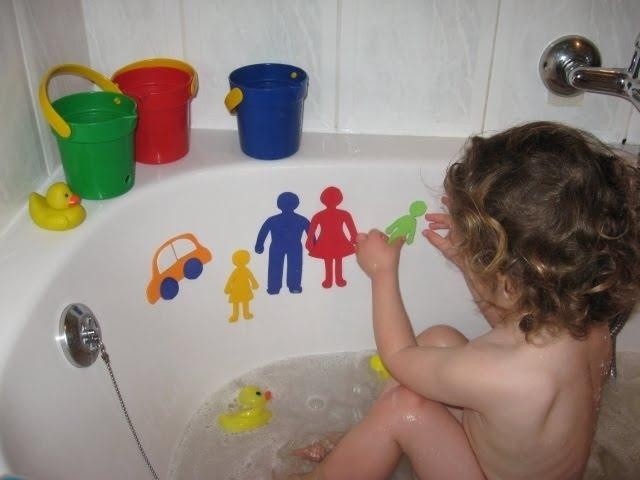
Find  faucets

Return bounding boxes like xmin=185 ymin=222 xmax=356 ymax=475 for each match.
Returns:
xmin=539 ymin=30 xmax=640 ymax=113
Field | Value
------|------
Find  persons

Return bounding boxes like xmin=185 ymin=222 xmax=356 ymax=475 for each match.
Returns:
xmin=270 ymin=121 xmax=640 ymax=480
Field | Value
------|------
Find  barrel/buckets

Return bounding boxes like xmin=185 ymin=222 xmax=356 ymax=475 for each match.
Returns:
xmin=111 ymin=58 xmax=198 ymax=165
xmin=38 ymin=62 xmax=137 ymax=200
xmin=224 ymin=64 xmax=308 ymax=160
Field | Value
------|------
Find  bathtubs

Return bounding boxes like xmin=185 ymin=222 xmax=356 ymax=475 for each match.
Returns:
xmin=0 ymin=127 xmax=640 ymax=480
